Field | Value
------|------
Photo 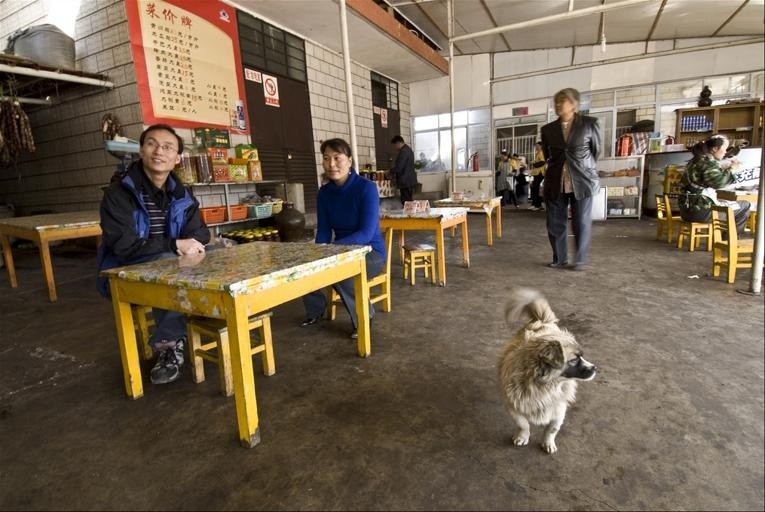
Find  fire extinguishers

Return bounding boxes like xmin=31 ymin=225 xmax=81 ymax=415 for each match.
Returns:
xmin=665 ymin=135 xmax=674 ymax=145
xmin=467 ymin=152 xmax=480 ymax=172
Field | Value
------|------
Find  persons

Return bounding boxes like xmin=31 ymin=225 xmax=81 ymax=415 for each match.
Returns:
xmin=418 ymin=152 xmax=432 ymax=171
xmin=540 ymin=89 xmax=603 ymax=272
xmin=298 ymin=137 xmax=387 ymax=338
xmin=96 ymin=123 xmax=212 ymax=384
xmin=387 ymin=135 xmax=417 ymax=210
xmin=677 ymin=135 xmax=751 ymax=242
xmin=495 ymin=141 xmax=546 ymax=212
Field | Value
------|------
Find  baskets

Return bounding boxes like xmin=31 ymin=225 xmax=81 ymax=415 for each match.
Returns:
xmin=224 ymin=205 xmax=248 ymax=221
xmin=247 ymin=203 xmax=273 ymax=218
xmin=200 ymin=207 xmax=225 ymax=224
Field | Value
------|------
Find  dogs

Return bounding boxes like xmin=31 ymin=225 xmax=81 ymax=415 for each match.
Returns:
xmin=500 ymin=288 xmax=597 ymax=455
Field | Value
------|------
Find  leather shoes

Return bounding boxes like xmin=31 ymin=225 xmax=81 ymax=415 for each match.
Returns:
xmin=350 ymin=328 xmax=358 ymax=339
xmin=300 ymin=314 xmax=324 ymax=328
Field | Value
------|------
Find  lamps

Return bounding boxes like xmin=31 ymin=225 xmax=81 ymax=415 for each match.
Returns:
xmin=600 ymin=10 xmax=607 ymax=55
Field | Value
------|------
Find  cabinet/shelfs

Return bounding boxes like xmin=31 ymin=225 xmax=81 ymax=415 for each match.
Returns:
xmin=595 ymin=155 xmax=645 ymax=220
xmin=673 ymin=101 xmax=765 ymax=149
xmin=181 ymin=178 xmax=290 ymax=237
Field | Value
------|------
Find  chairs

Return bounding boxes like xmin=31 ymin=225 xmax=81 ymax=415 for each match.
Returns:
xmin=323 ymin=227 xmax=394 ymax=318
xmin=710 ymin=207 xmax=755 ymax=283
xmin=654 ymin=194 xmax=684 ymax=242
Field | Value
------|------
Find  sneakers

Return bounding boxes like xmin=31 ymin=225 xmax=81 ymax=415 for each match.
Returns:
xmin=149 ymin=338 xmax=184 ymax=385
xmin=528 ymin=206 xmax=543 ymax=211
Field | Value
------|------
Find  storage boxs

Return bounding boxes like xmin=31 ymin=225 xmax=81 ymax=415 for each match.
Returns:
xmin=194 ymin=123 xmax=263 ymax=185
xmin=661 ymin=143 xmax=684 ymax=152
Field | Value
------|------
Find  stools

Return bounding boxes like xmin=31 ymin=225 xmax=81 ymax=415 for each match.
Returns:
xmin=676 ymin=217 xmax=714 ymax=252
xmin=186 ymin=312 xmax=279 ymax=397
xmin=130 ymin=297 xmax=155 ymax=362
xmin=742 ymin=210 xmax=757 ymax=234
xmin=402 ymin=242 xmax=438 ymax=286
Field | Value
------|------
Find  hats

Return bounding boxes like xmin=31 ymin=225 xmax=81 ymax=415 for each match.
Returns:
xmin=561 ymin=87 xmax=580 ymax=105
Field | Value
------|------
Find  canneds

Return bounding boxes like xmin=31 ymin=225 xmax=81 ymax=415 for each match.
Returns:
xmin=359 ymin=169 xmax=385 ymax=181
xmin=220 ymin=225 xmax=282 ymax=242
